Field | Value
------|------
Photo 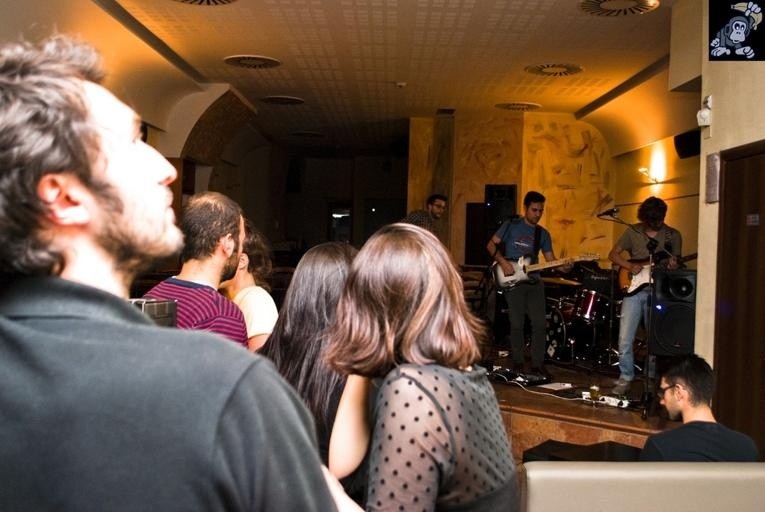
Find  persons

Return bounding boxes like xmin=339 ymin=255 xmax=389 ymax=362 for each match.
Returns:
xmin=313 ymin=220 xmax=519 ymax=511
xmin=266 ymin=238 xmax=361 ymax=469
xmin=398 ymin=192 xmax=448 ymax=235
xmin=485 ymin=191 xmax=576 ymax=379
xmin=608 ymin=197 xmax=682 ymax=395
xmin=0 ymin=21 xmax=338 ymax=511
xmin=218 ymin=216 xmax=280 ymax=352
xmin=143 ymin=191 xmax=250 ymax=347
xmin=638 ymin=353 xmax=761 ymax=462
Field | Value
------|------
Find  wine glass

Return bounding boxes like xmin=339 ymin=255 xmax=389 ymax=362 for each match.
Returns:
xmin=589 ymin=383 xmax=601 ymax=411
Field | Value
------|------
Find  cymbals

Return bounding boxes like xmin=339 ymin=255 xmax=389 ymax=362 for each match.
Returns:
xmin=541 ymin=276 xmax=582 ymax=286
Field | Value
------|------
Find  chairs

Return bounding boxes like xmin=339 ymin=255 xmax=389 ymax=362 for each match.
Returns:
xmin=519 ymin=461 xmax=764 ymax=511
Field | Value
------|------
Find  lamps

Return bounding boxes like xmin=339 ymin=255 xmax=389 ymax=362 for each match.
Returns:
xmin=638 ymin=168 xmax=659 ymax=184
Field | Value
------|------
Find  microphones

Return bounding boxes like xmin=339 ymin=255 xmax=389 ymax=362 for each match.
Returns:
xmin=500 ymin=213 xmax=520 ymax=222
xmin=597 ymin=207 xmax=619 ymax=218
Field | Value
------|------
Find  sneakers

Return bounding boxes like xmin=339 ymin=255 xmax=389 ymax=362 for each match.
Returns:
xmin=612 ymin=378 xmax=630 ymax=395
xmin=513 ymin=363 xmax=523 ymax=372
xmin=532 ymin=365 xmax=553 ymax=377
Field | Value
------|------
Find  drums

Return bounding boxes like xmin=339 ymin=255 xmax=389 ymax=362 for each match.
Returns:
xmin=545 ymin=296 xmax=596 ymax=363
xmin=571 ymin=287 xmax=612 ymax=325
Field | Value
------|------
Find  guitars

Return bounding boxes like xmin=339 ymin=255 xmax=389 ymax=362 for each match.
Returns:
xmin=490 ymin=252 xmax=600 ymax=295
xmin=617 ymin=251 xmax=697 ymax=297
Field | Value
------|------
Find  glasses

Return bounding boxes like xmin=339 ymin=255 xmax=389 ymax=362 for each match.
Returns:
xmin=657 ymin=385 xmax=677 ymax=398
xmin=432 ymin=203 xmax=448 ymax=211
